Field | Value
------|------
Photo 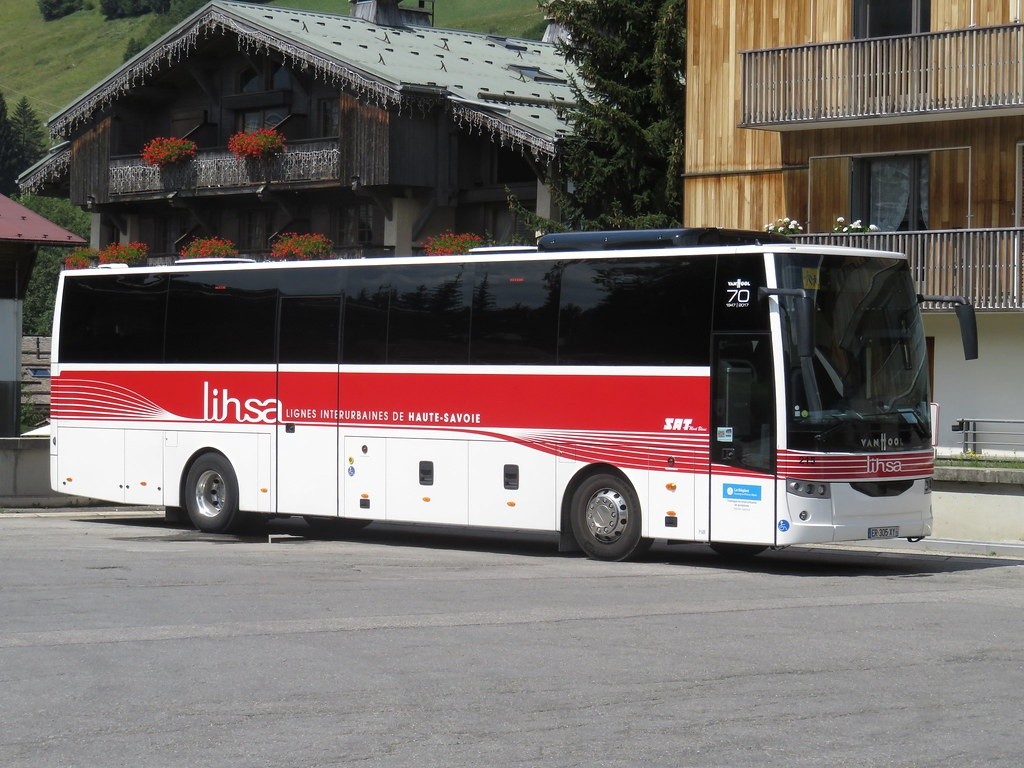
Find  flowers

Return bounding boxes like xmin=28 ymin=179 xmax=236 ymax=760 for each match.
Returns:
xmin=66 ymin=241 xmax=149 ymax=269
xmin=763 ymin=218 xmax=804 ymax=235
xmin=832 ymin=216 xmax=878 ymax=232
xmin=178 ymin=236 xmax=239 ymax=259
xmin=229 ymin=128 xmax=287 ymax=160
xmin=419 ymin=229 xmax=485 ymax=256
xmin=140 ymin=137 xmax=199 ymax=167
xmin=271 ymin=233 xmax=337 ymax=259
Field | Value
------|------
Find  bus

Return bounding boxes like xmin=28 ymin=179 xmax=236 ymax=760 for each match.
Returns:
xmin=50 ymin=226 xmax=981 ymax=562
